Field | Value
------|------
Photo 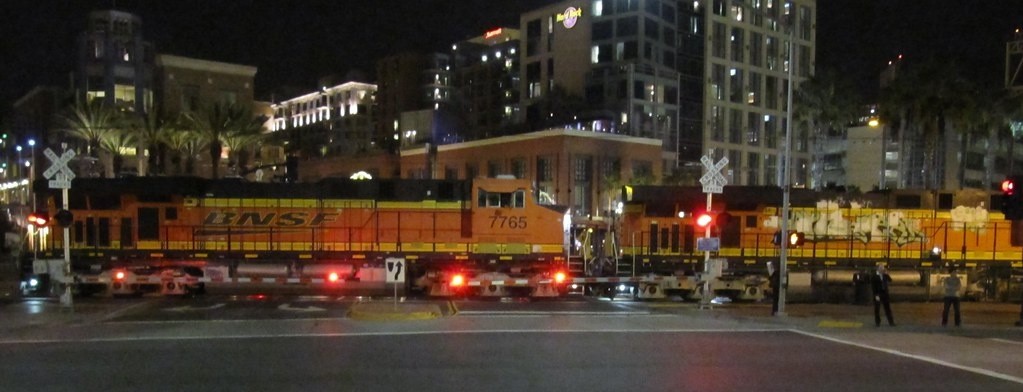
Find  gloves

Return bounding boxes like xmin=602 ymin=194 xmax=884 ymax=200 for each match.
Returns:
xmin=876 ymin=296 xmax=880 ymax=301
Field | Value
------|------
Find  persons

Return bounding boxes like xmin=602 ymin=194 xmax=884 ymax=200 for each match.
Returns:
xmin=941 ymin=268 xmax=963 ymax=326
xmin=768 ymin=258 xmax=789 ymax=315
xmin=870 ymin=264 xmax=897 ymax=327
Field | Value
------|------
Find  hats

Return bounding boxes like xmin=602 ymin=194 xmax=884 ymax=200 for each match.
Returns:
xmin=949 ymin=268 xmax=956 ymax=273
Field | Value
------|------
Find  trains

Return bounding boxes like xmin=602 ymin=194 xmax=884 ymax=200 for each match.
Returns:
xmin=619 ymin=186 xmax=1022 ymax=311
xmin=26 ymin=176 xmax=572 ymax=296
